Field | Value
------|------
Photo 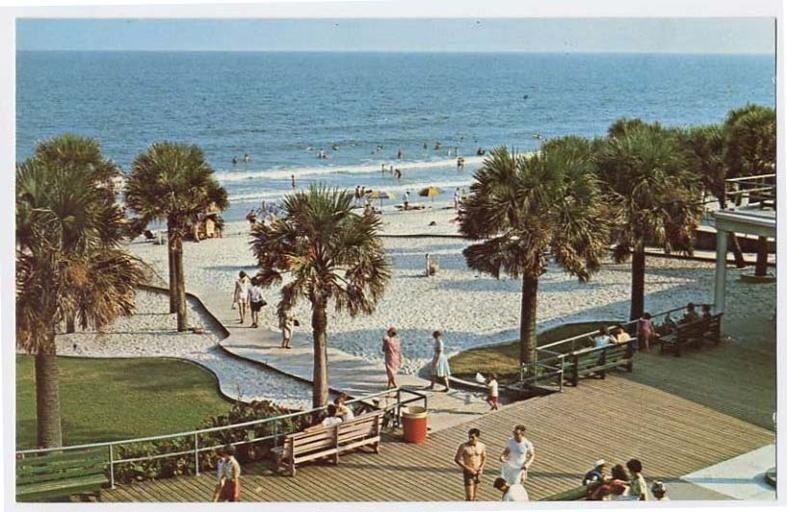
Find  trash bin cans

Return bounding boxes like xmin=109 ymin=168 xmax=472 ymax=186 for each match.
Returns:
xmin=402 ymin=406 xmax=428 ymax=443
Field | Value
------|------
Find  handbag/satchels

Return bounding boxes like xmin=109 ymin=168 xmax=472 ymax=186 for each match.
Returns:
xmin=255 ymin=299 xmax=267 ymax=308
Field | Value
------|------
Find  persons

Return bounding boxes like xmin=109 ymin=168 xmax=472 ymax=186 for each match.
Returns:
xmin=277 ymin=291 xmax=299 ymax=349
xmin=381 ymin=327 xmax=404 ymax=390
xmin=423 ymin=330 xmax=452 ymax=393
xmin=484 ymin=373 xmax=500 ymax=411
xmin=554 ymin=300 xmax=713 ymax=373
xmin=492 ymin=478 xmax=529 ymax=502
xmin=500 ymin=424 xmax=536 ymax=484
xmin=320 ymin=390 xmax=372 ymax=458
xmin=454 ymin=427 xmax=488 ymax=501
xmin=246 ymin=276 xmax=268 ymax=328
xmin=218 ymin=444 xmax=241 ymax=502
xmin=211 ymin=447 xmax=227 ymax=502
xmin=233 ymin=270 xmax=252 ymax=323
xmin=582 ymin=456 xmax=673 ymax=500
xmin=228 ymin=132 xmax=489 ymax=278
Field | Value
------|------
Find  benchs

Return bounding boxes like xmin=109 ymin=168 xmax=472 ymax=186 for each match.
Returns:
xmin=269 ymin=409 xmax=382 ymax=478
xmin=556 ymin=336 xmax=638 ymax=388
xmin=655 ymin=310 xmax=725 ymax=357
xmin=16 ymin=445 xmax=113 ymax=503
